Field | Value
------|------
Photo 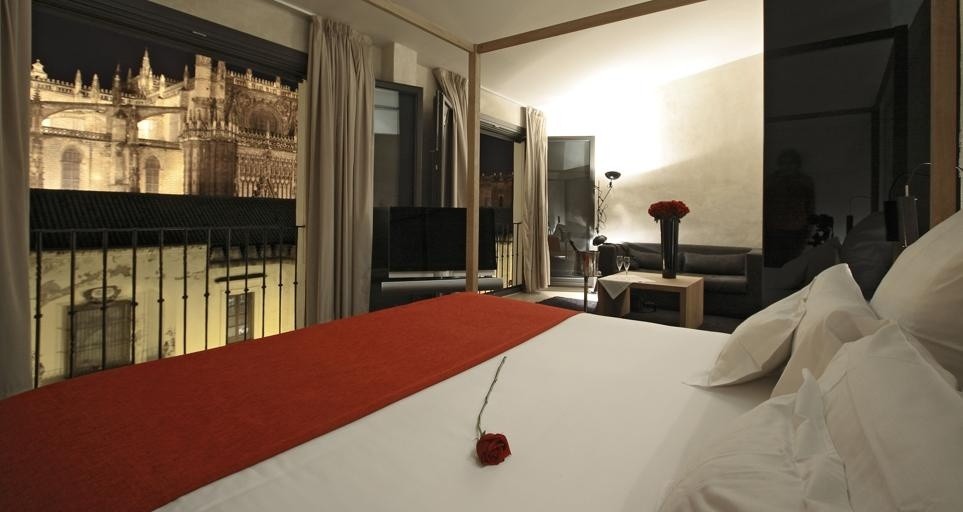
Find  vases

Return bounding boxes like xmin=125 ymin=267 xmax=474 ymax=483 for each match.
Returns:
xmin=659 ymin=218 xmax=679 ymax=279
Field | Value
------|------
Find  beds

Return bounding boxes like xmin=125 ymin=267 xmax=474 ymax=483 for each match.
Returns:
xmin=0 ymin=288 xmax=772 ymax=512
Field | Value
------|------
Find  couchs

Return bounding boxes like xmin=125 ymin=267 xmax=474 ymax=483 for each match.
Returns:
xmin=597 ymin=242 xmax=764 ymax=318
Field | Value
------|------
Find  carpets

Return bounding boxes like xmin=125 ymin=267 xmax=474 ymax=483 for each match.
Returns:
xmin=536 ymin=295 xmax=745 ymax=334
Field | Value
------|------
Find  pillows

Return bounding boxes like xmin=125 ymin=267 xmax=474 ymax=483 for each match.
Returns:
xmin=768 ymin=260 xmax=881 ymax=406
xmin=815 ymin=322 xmax=961 ymax=512
xmin=626 ymin=250 xmax=745 ymax=275
xmin=665 ymin=371 xmax=855 ymax=512
xmin=680 ymin=275 xmax=821 ymax=400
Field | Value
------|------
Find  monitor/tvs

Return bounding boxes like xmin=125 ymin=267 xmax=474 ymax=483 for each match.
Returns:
xmin=387 ymin=205 xmax=497 ymax=279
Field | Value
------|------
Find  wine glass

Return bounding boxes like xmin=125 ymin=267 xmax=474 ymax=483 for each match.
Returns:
xmin=616 ymin=255 xmax=631 ymax=275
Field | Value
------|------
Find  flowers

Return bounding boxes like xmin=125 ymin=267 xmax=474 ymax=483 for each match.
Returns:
xmin=470 ymin=355 xmax=515 ymax=469
xmin=647 ymin=199 xmax=689 ymax=222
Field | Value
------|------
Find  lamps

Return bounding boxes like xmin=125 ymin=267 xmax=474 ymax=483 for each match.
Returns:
xmin=591 ymin=169 xmax=620 ymax=246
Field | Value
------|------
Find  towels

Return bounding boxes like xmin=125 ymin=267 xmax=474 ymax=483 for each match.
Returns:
xmin=593 ymin=274 xmax=656 ymax=301
